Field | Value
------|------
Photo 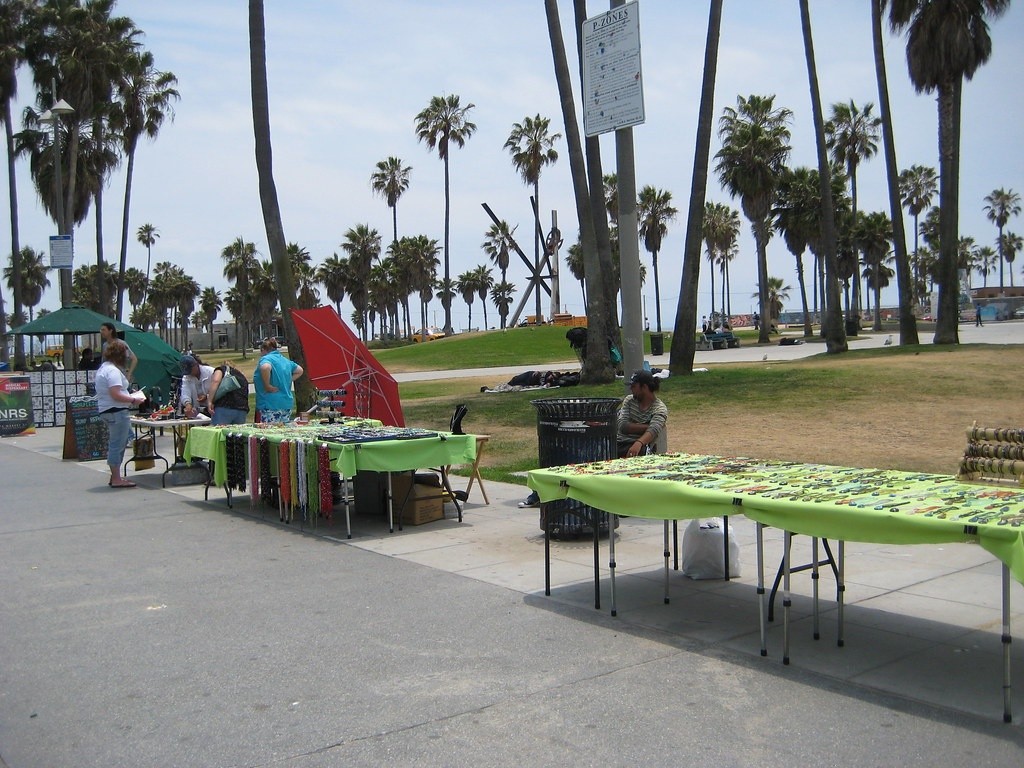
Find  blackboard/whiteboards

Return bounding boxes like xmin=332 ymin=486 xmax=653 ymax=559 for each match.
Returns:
xmin=62 ymin=395 xmax=110 ymax=462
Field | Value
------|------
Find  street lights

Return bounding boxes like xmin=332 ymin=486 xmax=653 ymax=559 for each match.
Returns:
xmin=36 ymin=98 xmax=82 ymax=371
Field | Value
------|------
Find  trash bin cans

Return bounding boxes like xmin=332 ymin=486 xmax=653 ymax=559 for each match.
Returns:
xmin=845 ymin=319 xmax=858 ymax=337
xmin=650 ymin=333 xmax=664 ymax=356
xmin=530 ymin=397 xmax=625 ymax=539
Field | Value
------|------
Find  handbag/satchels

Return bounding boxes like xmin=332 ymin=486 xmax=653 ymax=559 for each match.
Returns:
xmin=212 ymin=366 xmax=242 ymax=403
xmin=682 ymin=518 xmax=742 ymax=580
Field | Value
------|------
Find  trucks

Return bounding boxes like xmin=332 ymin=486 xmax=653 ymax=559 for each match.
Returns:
xmin=518 ymin=315 xmax=546 ymax=326
xmin=544 ymin=314 xmax=587 ymax=328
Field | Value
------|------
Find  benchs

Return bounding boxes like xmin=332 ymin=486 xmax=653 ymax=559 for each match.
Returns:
xmin=696 ymin=333 xmax=740 ymax=351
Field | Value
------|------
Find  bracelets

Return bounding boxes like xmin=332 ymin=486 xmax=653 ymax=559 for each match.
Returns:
xmin=962 ymin=422 xmax=1024 ymax=475
xmin=131 ymin=398 xmax=135 ymax=404
xmin=636 ymin=440 xmax=644 ymax=447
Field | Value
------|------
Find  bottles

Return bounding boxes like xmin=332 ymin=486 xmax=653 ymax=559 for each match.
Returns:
xmin=159 ymin=405 xmax=177 ymax=420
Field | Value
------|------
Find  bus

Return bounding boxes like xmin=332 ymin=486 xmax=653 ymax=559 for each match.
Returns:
xmin=47 ymin=345 xmax=81 ymax=357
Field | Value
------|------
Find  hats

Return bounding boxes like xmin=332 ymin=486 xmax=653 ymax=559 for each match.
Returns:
xmin=180 ymin=355 xmax=194 ymax=377
xmin=627 ymin=369 xmax=654 ymax=385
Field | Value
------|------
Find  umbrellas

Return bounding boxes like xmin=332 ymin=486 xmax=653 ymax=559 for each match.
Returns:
xmin=124 ymin=330 xmax=183 ymax=413
xmin=3 ymin=304 xmax=141 ymax=396
xmin=288 ymin=305 xmax=405 ymax=428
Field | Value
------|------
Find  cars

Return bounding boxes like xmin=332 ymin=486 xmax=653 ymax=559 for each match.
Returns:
xmin=1016 ymin=306 xmax=1024 ymax=319
xmin=923 ymin=315 xmax=932 ymax=321
xmin=412 ymin=327 xmax=446 ymax=343
xmin=254 ymin=336 xmax=284 ymax=349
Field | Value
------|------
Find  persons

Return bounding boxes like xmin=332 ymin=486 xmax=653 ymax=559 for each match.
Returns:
xmin=254 ymin=337 xmax=303 ymax=423
xmin=702 ymin=316 xmax=707 ymax=332
xmin=752 ymin=312 xmax=758 ymax=330
xmin=705 ymin=326 xmax=713 ymax=333
xmin=518 ymin=370 xmax=668 ymax=508
xmin=78 ymin=348 xmax=93 ymax=370
xmin=95 ymin=342 xmax=144 ymax=488
xmin=180 ymin=355 xmax=215 ymax=417
xmin=976 ymin=303 xmax=982 ymax=326
xmin=723 ymin=324 xmax=729 ymax=332
xmin=645 ymin=318 xmax=649 ymax=331
xmin=204 ymin=359 xmax=249 ymax=485
xmin=100 ymin=323 xmax=138 ymax=447
xmin=714 ymin=325 xmax=722 ymax=333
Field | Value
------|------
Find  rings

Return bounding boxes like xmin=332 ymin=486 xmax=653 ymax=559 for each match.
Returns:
xmin=630 ymin=453 xmax=632 ymax=456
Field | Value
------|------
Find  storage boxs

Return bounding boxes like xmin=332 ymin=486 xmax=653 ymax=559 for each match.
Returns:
xmin=388 ymin=472 xmax=446 ymax=526
xmin=352 ymin=470 xmax=379 ymax=506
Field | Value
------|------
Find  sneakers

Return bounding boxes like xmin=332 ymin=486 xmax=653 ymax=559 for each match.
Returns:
xmin=518 ymin=492 xmax=539 ymax=508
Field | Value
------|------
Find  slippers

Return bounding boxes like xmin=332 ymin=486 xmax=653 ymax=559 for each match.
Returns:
xmin=109 ymin=479 xmax=136 ymax=487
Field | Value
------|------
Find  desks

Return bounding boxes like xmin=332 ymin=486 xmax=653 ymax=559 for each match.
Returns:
xmin=124 ymin=414 xmax=214 ymax=488
xmin=182 ymin=416 xmax=491 ymax=539
xmin=528 ymin=451 xmax=1024 ymax=723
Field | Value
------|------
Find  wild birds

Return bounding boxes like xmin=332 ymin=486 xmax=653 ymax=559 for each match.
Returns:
xmin=763 ymin=353 xmax=768 ymax=360
xmin=884 ymin=335 xmax=893 ymax=346
